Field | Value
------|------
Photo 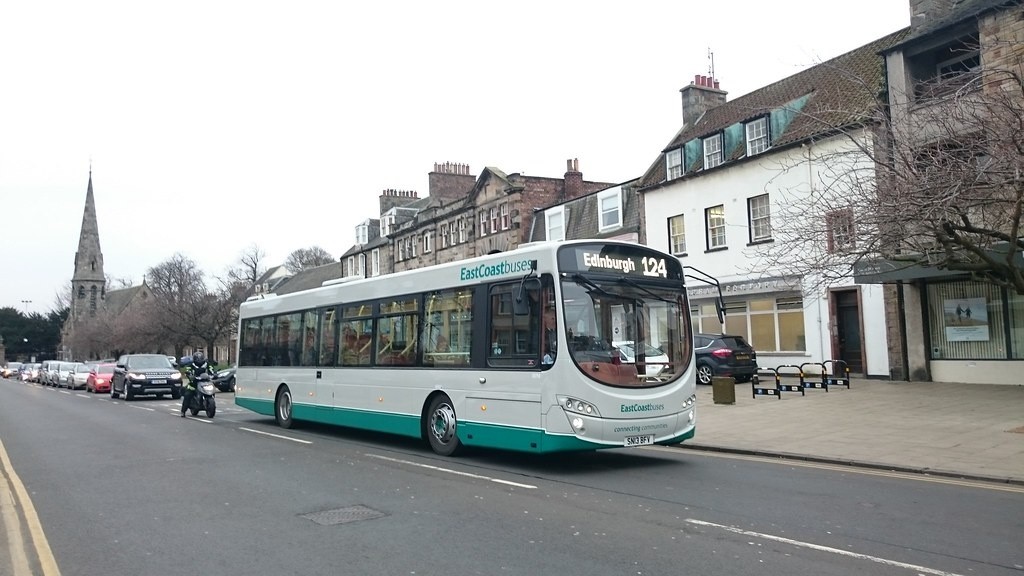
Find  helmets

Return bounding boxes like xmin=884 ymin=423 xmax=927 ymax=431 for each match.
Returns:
xmin=193 ymin=352 xmax=204 ymax=366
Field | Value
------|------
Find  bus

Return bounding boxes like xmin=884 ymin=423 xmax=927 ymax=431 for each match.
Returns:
xmin=233 ymin=239 xmax=728 ymax=457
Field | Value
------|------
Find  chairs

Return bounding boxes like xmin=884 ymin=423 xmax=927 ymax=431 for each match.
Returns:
xmin=359 ymin=355 xmax=409 ymax=364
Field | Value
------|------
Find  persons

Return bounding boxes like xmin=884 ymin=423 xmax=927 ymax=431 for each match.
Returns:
xmin=379 ymin=334 xmax=390 ymax=350
xmin=956 ymin=304 xmax=972 ymax=318
xmin=545 ymin=309 xmax=556 ymax=352
xmin=181 ymin=351 xmax=215 ymax=417
xmin=433 ymin=335 xmax=452 ymax=359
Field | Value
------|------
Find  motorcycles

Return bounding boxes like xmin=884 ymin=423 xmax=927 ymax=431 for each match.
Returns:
xmin=184 ymin=368 xmax=222 ymax=418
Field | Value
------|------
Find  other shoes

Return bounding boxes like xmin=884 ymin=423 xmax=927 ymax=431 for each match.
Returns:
xmin=181 ymin=411 xmax=185 ymax=417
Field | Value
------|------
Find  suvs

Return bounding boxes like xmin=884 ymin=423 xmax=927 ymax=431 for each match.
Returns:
xmin=110 ymin=353 xmax=183 ymax=401
xmin=694 ymin=332 xmax=758 ymax=384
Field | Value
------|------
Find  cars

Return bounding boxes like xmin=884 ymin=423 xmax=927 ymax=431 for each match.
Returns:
xmin=52 ymin=362 xmax=84 ymax=388
xmin=0 ymin=360 xmax=66 ymax=387
xmin=86 ymin=363 xmax=117 ymax=394
xmin=212 ymin=366 xmax=236 ymax=392
xmin=67 ymin=363 xmax=92 ymax=389
